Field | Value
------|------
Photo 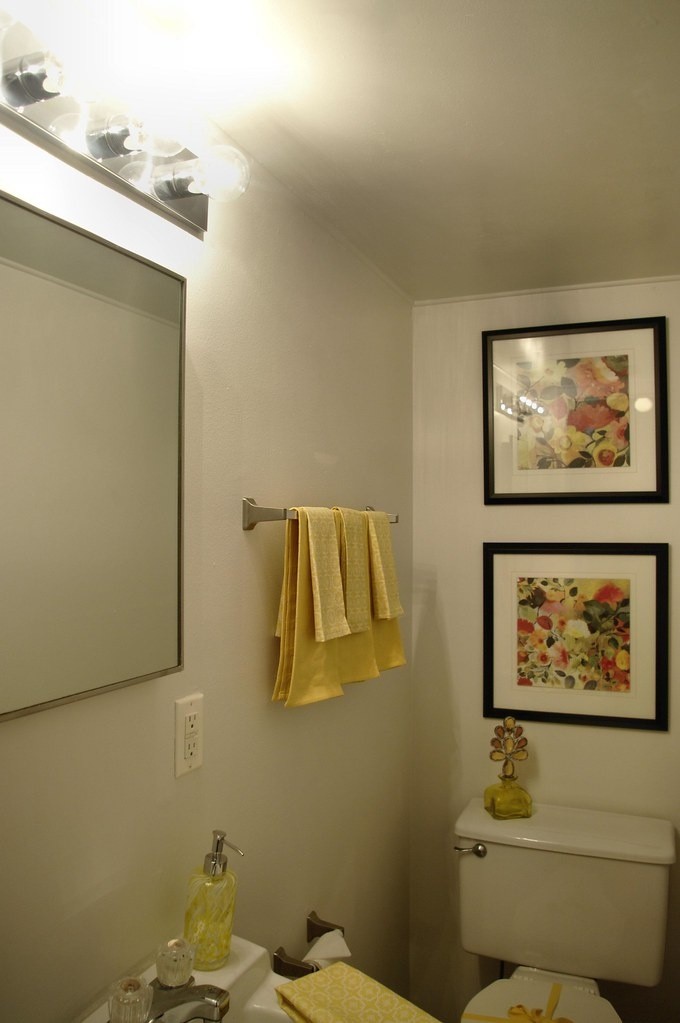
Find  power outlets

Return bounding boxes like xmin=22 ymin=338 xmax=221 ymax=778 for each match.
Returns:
xmin=174 ymin=694 xmax=206 ymax=777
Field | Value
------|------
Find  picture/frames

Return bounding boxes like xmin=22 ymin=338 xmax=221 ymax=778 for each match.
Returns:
xmin=482 ymin=541 xmax=671 ymax=731
xmin=480 ymin=316 xmax=670 ymax=506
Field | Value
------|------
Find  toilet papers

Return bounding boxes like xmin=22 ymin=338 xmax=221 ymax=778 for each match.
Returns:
xmin=301 ymin=928 xmax=351 ymax=970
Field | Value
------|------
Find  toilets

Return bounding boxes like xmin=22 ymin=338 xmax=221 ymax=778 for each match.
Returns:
xmin=451 ymin=797 xmax=677 ymax=1023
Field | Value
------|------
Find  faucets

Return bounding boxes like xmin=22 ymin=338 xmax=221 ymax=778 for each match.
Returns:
xmin=145 ymin=984 xmax=230 ymax=1023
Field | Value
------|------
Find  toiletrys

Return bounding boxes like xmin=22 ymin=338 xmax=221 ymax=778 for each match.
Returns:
xmin=183 ymin=829 xmax=244 ymax=972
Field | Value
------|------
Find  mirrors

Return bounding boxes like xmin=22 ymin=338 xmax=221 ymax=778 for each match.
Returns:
xmin=0 ymin=185 xmax=188 ymax=727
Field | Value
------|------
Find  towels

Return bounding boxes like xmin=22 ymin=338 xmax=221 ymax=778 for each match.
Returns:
xmin=275 ymin=961 xmax=442 ymax=1023
xmin=270 ymin=506 xmax=407 ymax=709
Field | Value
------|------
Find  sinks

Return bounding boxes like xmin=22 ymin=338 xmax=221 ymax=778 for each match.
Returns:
xmin=80 ymin=924 xmax=292 ymax=1023
